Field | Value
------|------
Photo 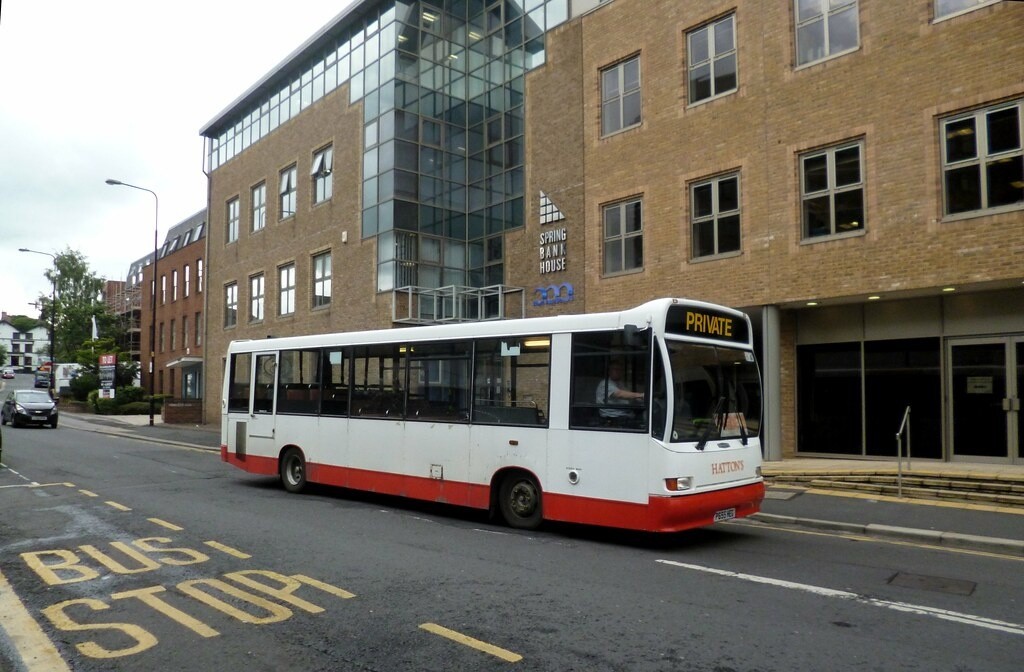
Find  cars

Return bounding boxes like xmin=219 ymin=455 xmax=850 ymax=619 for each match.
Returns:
xmin=35 ymin=371 xmax=49 ymax=388
xmin=1 ymin=390 xmax=58 ymax=429
xmin=2 ymin=369 xmax=16 ymax=379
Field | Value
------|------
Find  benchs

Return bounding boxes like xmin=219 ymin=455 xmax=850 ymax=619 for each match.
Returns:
xmin=472 ymin=399 xmax=546 ymax=424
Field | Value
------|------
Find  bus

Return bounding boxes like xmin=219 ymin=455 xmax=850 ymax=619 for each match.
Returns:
xmin=220 ymin=297 xmax=766 ymax=532
xmin=49 ymin=361 xmax=152 ymax=400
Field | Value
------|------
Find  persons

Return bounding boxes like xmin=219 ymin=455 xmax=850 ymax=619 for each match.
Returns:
xmin=595 ymin=358 xmax=645 ymax=426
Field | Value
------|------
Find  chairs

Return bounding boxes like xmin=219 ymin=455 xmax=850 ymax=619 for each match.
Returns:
xmin=243 ymin=384 xmax=346 ymax=413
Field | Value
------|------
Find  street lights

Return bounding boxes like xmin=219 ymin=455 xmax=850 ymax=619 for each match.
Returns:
xmin=105 ymin=179 xmax=158 ymax=427
xmin=19 ymin=248 xmax=56 ymax=398
